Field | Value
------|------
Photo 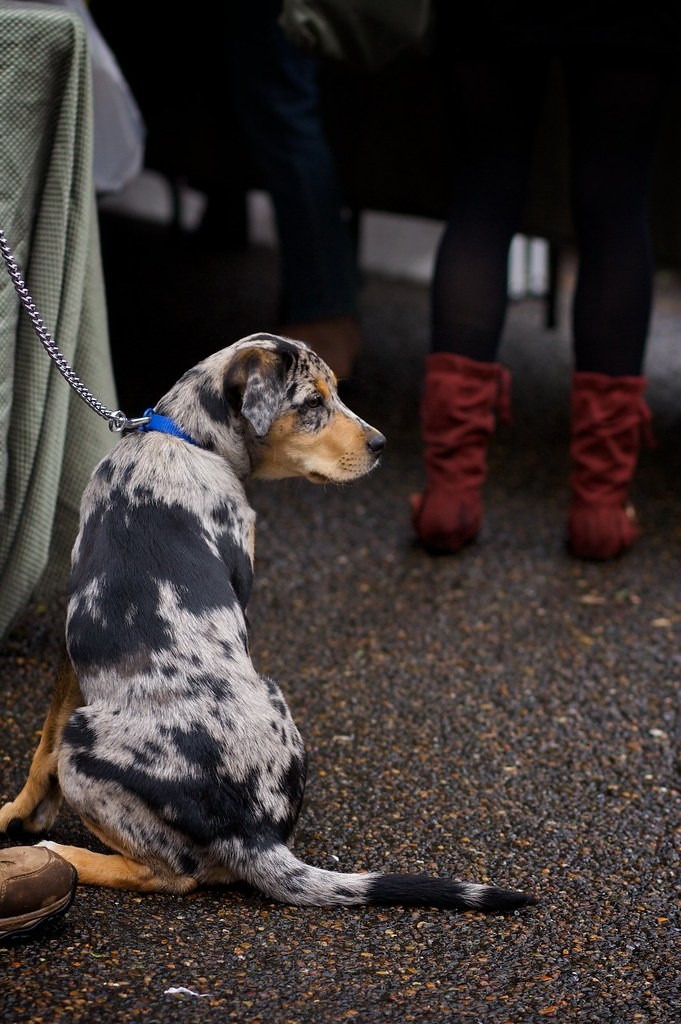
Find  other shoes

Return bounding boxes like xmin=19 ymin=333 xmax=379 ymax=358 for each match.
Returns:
xmin=295 ymin=317 xmax=362 ymax=397
xmin=0 ymin=846 xmax=78 ymax=943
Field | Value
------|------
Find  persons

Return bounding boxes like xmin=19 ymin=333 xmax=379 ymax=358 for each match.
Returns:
xmin=413 ymin=1 xmax=681 ymax=559
xmin=1 ymin=0 xmax=433 ymax=391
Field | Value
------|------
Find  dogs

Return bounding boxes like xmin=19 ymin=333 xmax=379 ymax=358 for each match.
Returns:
xmin=0 ymin=330 xmax=546 ymax=915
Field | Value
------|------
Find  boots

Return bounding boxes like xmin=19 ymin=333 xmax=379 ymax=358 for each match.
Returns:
xmin=568 ymin=372 xmax=655 ymax=562
xmin=410 ymin=353 xmax=512 ymax=555
